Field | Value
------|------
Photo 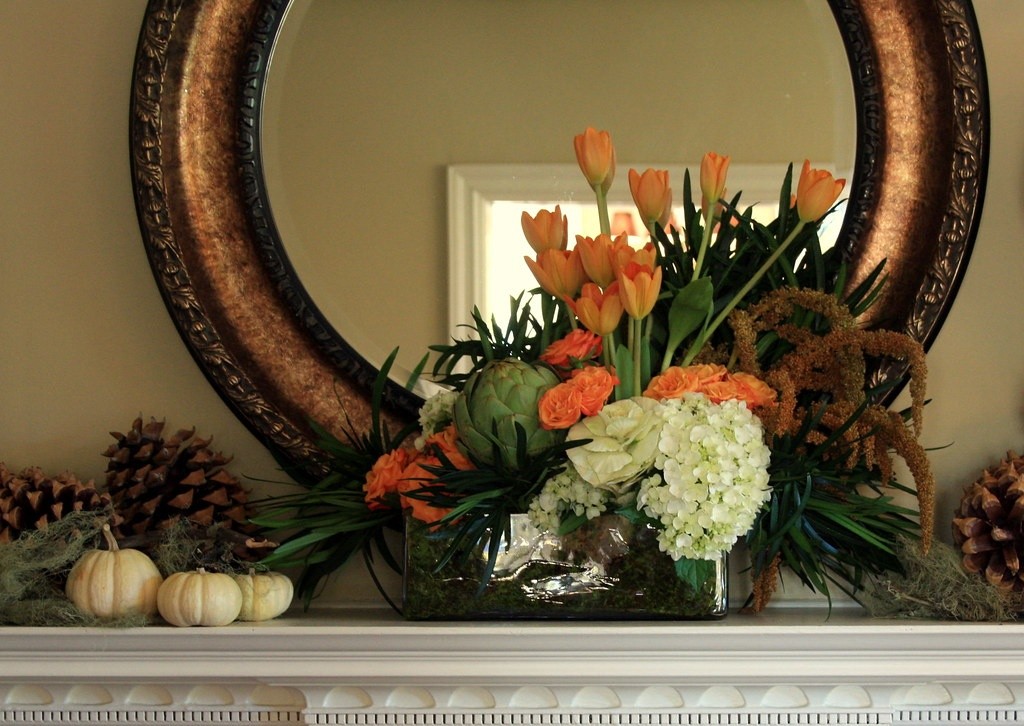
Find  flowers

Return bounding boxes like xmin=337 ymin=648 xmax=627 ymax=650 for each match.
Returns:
xmin=220 ymin=126 xmax=957 ymax=620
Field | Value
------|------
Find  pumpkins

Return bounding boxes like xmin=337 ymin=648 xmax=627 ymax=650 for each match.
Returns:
xmin=156 ymin=567 xmax=242 ymax=627
xmin=65 ymin=524 xmax=163 ymax=621
xmin=228 ymin=568 xmax=293 ymax=622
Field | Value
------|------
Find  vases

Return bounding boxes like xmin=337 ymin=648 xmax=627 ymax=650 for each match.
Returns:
xmin=401 ymin=512 xmax=729 ymax=623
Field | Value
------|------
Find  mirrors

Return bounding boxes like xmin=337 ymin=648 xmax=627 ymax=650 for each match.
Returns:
xmin=129 ymin=0 xmax=992 ymax=528
